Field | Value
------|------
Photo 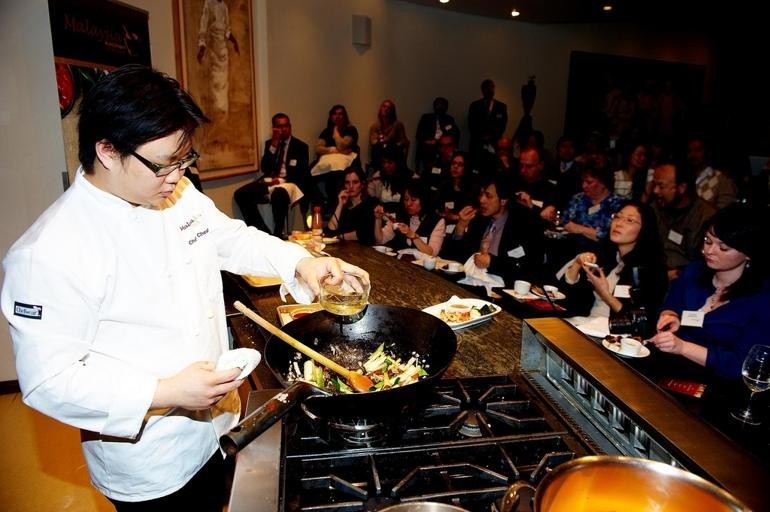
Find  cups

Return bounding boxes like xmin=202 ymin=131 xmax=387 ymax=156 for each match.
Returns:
xmin=514 ymin=280 xmax=532 ymax=295
xmin=550 ymin=209 xmax=565 ymax=225
xmin=423 ymin=259 xmax=437 ymax=271
xmin=392 ymin=223 xmax=400 ymax=231
xmin=543 ymin=284 xmax=558 ymax=294
xmin=621 ymin=338 xmax=642 ymax=356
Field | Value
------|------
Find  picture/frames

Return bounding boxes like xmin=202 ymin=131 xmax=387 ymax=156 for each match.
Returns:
xmin=172 ymin=0 xmax=260 ymax=180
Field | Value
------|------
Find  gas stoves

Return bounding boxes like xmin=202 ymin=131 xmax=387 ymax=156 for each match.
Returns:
xmin=275 ymin=364 xmax=624 ymax=512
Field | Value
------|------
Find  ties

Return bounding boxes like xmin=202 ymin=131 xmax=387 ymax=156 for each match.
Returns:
xmin=273 ymin=140 xmax=286 ymax=176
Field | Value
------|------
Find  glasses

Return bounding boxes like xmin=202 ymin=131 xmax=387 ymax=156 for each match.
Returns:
xmin=131 ymin=148 xmax=200 ymax=177
xmin=611 ymin=213 xmax=641 ymax=225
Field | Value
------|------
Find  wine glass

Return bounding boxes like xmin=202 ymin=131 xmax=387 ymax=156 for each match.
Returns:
xmin=730 ymin=344 xmax=770 ymax=426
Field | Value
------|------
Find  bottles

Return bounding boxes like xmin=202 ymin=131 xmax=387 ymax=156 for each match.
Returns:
xmin=313 ymin=206 xmax=323 ymax=244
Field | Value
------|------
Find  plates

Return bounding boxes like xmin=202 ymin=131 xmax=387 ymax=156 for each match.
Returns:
xmin=323 ymin=237 xmax=339 ymax=244
xmin=421 ymin=298 xmax=503 ymax=332
xmin=372 ymin=246 xmax=394 ymax=252
xmin=438 ymin=263 xmax=463 ymax=274
xmin=532 ymin=287 xmax=567 ymax=301
xmin=241 ymin=274 xmax=282 ymax=288
xmin=602 ymin=338 xmax=651 ymax=360
xmin=502 ymin=289 xmax=541 ymax=300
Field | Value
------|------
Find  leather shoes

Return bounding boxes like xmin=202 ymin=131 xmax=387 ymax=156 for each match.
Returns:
xmin=274 ymin=233 xmax=288 ymax=240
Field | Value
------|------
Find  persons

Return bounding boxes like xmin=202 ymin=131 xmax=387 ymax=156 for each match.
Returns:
xmin=233 ymin=113 xmax=309 ymax=237
xmin=0 ymin=65 xmax=371 ymax=511
xmin=327 ymin=165 xmax=375 ymax=247
xmin=310 ymin=104 xmax=361 ymax=219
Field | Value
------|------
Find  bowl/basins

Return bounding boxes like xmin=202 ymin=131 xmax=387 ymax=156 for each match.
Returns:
xmin=378 ymin=500 xmax=469 ymax=512
xmin=320 ymin=272 xmax=371 ymax=316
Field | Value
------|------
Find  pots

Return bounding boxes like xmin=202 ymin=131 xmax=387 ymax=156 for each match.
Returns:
xmin=500 ymin=453 xmax=753 ymax=512
xmin=218 ymin=303 xmax=457 ymax=458
